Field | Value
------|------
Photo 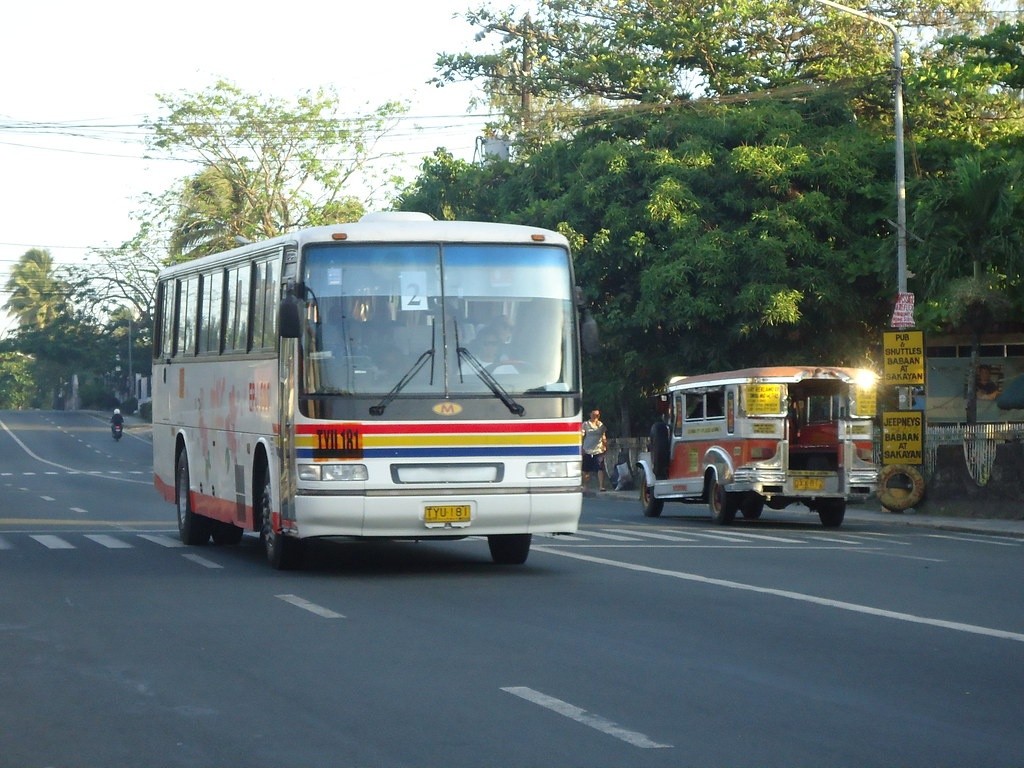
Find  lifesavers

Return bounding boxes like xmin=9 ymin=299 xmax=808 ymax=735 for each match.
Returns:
xmin=875 ymin=464 xmax=925 ymax=511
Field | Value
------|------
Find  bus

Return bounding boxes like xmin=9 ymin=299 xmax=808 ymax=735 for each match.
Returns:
xmin=150 ymin=210 xmax=585 ymax=571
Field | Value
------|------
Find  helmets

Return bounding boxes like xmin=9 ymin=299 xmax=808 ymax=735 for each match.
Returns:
xmin=114 ymin=408 xmax=120 ymax=414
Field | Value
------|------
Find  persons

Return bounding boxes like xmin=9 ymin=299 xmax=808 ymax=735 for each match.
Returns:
xmin=582 ymin=409 xmax=607 ymax=491
xmin=110 ymin=409 xmax=123 ymax=437
xmin=976 ymin=366 xmax=1000 ymax=400
xmin=315 ymin=308 xmax=525 ymax=375
xmin=610 ymin=453 xmax=634 ymax=491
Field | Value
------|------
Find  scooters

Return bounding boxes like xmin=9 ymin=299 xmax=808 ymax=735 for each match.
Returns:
xmin=111 ymin=419 xmax=124 ymax=441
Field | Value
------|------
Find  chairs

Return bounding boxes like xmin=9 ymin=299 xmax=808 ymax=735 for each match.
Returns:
xmin=307 ymin=317 xmax=512 ymax=392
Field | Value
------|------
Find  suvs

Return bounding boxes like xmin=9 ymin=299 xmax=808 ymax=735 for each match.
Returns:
xmin=637 ymin=364 xmax=880 ymax=528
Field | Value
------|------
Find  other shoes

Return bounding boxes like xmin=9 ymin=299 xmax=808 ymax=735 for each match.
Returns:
xmin=600 ymin=487 xmax=606 ymax=492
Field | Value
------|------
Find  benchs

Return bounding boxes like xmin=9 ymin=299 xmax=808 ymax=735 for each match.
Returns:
xmin=788 ymin=420 xmax=843 ymax=455
